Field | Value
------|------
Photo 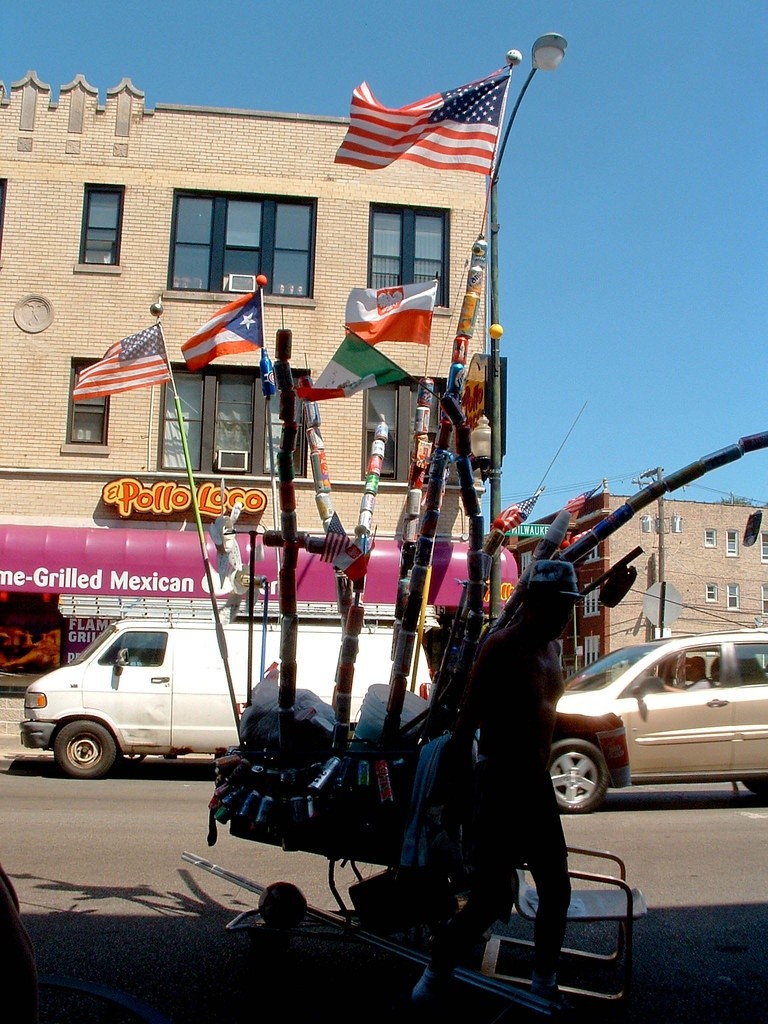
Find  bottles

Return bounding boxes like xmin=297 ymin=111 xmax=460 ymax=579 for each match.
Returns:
xmin=260 ymin=347 xmax=275 ymax=395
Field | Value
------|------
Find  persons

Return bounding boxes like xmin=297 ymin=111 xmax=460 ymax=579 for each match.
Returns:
xmin=660 ymin=656 xmax=712 ymax=692
xmin=407 ymin=560 xmax=580 ymax=1024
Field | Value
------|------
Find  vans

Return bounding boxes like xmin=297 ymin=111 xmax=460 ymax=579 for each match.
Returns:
xmin=20 ymin=618 xmax=435 ymax=783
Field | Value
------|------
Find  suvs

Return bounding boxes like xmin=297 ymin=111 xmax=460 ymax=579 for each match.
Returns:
xmin=550 ymin=626 xmax=768 ymax=812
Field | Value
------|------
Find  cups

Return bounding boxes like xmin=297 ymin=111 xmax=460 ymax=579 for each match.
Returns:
xmin=596 ymin=726 xmax=631 ymax=787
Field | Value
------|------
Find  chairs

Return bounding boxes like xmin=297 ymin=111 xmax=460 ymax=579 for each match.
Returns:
xmin=480 ymin=844 xmax=649 ymax=1003
xmin=710 ymin=656 xmax=720 ymax=682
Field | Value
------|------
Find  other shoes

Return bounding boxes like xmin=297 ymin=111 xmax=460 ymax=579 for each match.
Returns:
xmin=412 ymin=963 xmax=442 ymax=1004
xmin=531 ymin=984 xmax=576 ymax=1019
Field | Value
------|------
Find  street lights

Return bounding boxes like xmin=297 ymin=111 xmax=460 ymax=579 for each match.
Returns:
xmin=639 ymin=466 xmax=667 ymax=640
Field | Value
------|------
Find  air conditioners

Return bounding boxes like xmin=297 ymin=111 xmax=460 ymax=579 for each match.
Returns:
xmin=217 ymin=449 xmax=249 ymax=472
xmin=228 ymin=274 xmax=256 ymax=293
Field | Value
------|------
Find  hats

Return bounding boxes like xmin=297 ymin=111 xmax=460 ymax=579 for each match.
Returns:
xmin=525 ymin=559 xmax=586 ymax=600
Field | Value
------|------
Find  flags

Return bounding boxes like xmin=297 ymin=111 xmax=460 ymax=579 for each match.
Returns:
xmin=72 ymin=67 xmax=511 ymax=403
